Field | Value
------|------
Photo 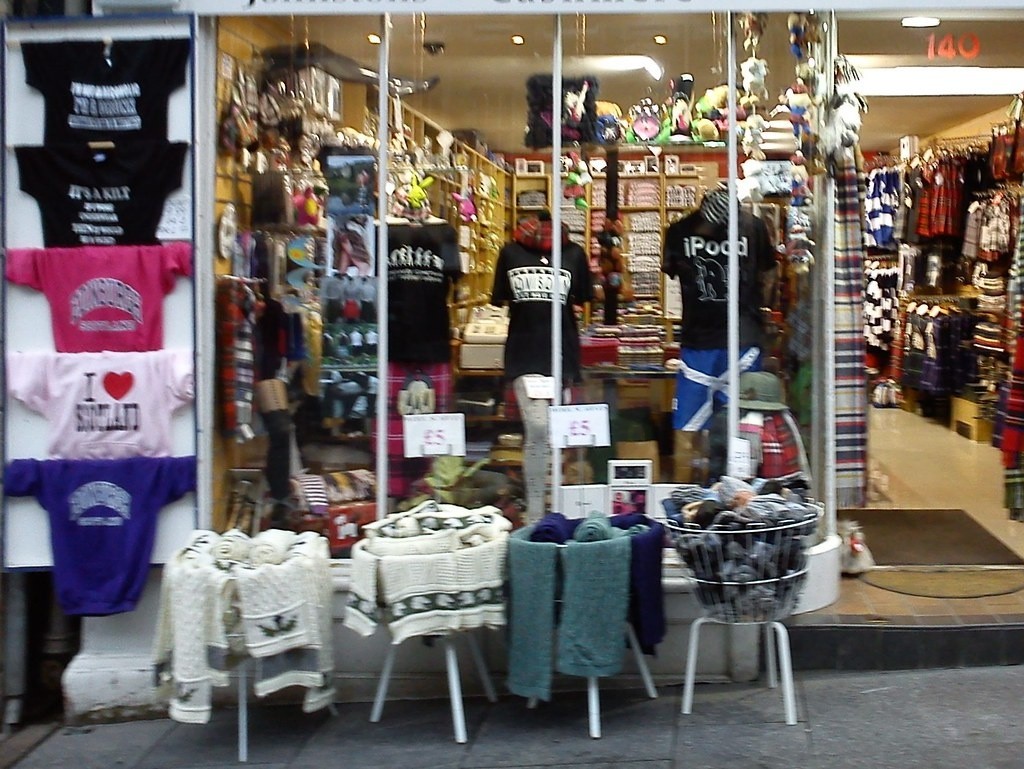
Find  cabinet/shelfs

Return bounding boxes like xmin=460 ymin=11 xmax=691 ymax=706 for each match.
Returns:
xmin=337 ymin=80 xmax=704 ymax=424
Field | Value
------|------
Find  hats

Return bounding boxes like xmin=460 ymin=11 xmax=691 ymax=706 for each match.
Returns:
xmin=393 ymin=174 xmax=434 ymax=221
xmin=216 ymin=100 xmax=261 ymax=157
xmin=721 ymin=371 xmax=791 ymax=412
xmin=513 ymin=209 xmax=570 ymax=250
xmin=701 ymin=188 xmax=742 ymax=227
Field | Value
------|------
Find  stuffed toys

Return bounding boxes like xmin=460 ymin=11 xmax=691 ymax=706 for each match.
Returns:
xmin=595 ymin=10 xmax=862 ymax=275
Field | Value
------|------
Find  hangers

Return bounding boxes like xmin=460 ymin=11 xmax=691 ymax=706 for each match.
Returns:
xmin=864 ymin=134 xmax=1024 ymax=318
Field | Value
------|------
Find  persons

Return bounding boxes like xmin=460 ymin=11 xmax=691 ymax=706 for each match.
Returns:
xmin=660 ymin=183 xmax=778 ymax=482
xmin=493 ymin=208 xmax=596 ymax=528
xmin=374 ymin=186 xmax=462 ymax=497
